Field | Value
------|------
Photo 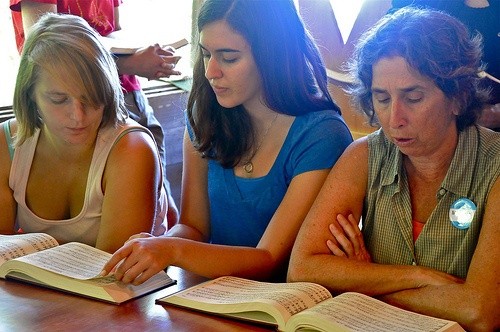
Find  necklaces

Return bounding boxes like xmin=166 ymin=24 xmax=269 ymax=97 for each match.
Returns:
xmin=240 ymin=112 xmax=279 ymax=173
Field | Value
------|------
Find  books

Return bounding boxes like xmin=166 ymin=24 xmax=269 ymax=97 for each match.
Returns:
xmin=0 ymin=232 xmax=465 ymax=332
xmin=98 ymin=29 xmax=189 ymax=54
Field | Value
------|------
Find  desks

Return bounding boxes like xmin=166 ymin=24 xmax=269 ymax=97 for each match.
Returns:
xmin=0 ymin=265 xmax=277 ymax=332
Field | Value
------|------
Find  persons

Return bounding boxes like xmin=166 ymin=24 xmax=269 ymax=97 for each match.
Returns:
xmin=8 ymin=0 xmax=500 ymax=230
xmin=0 ymin=13 xmax=168 ymax=254
xmin=99 ymin=0 xmax=354 ymax=285
xmin=287 ymin=7 xmax=500 ymax=332
